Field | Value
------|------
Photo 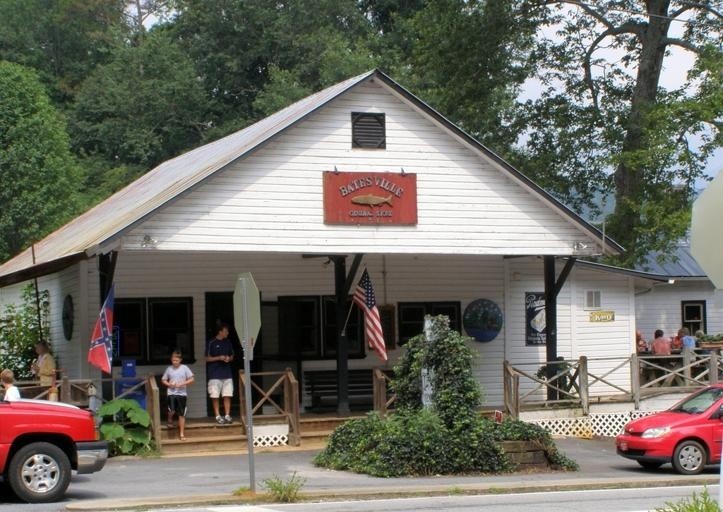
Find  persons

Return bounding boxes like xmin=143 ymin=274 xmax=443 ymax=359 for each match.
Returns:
xmin=31 ymin=340 xmax=56 ymax=386
xmin=636 ymin=328 xmax=705 ymax=355
xmin=0 ymin=368 xmax=21 ymax=401
xmin=205 ymin=322 xmax=235 ymax=423
xmin=161 ymin=349 xmax=195 ymax=441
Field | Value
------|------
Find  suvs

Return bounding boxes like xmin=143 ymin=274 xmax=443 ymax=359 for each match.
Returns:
xmin=1 ymin=399 xmax=107 ymax=503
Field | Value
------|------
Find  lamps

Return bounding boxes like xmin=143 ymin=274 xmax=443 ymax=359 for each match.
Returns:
xmin=141 ymin=235 xmax=158 ymax=248
xmin=574 ymin=240 xmax=588 ymax=250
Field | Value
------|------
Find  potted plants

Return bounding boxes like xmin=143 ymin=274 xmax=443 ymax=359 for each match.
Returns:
xmin=696 ymin=333 xmax=723 ymax=347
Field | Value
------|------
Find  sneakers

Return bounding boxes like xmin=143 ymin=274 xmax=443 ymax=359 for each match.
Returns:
xmin=223 ymin=416 xmax=233 ymax=424
xmin=215 ymin=416 xmax=224 ymax=424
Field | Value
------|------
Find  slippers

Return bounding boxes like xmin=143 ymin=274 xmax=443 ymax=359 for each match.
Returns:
xmin=180 ymin=436 xmax=186 ymax=442
xmin=167 ymin=422 xmax=173 ymax=428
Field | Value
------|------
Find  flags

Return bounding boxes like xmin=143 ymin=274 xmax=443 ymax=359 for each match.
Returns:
xmin=88 ymin=285 xmax=114 ymax=374
xmin=352 ymin=268 xmax=387 ymax=360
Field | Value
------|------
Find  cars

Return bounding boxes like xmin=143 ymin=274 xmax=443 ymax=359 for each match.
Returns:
xmin=616 ymin=383 xmax=723 ymax=475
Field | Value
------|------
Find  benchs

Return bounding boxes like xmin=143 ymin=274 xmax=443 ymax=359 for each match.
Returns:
xmin=646 ymin=365 xmax=706 ymax=387
xmin=303 ymin=369 xmax=394 ymax=413
